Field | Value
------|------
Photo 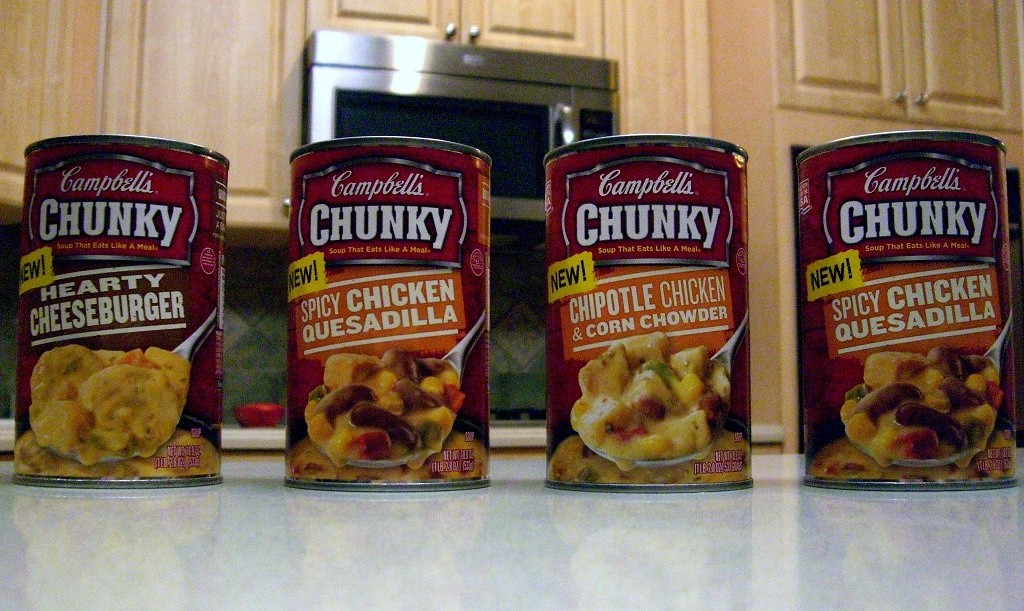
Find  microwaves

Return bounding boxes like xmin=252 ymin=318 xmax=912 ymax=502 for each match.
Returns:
xmin=299 ymin=28 xmax=620 ymax=234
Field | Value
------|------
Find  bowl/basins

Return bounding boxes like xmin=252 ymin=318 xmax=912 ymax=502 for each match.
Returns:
xmin=233 ymin=399 xmax=282 ymax=428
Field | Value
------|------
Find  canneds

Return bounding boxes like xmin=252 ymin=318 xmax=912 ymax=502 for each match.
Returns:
xmin=12 ymin=133 xmax=1017 ymax=492
